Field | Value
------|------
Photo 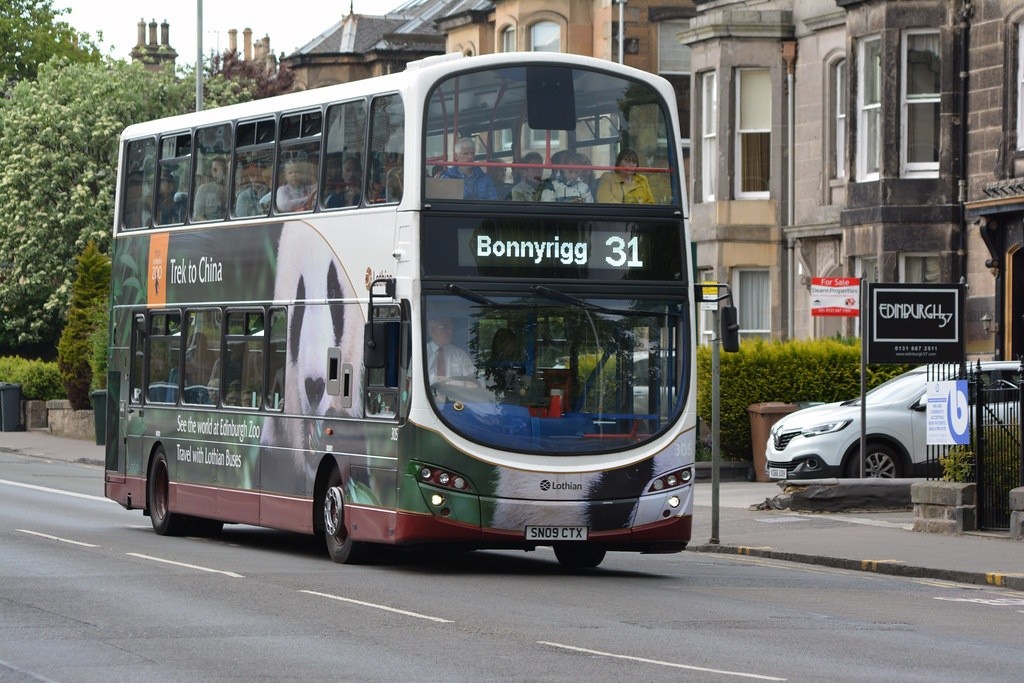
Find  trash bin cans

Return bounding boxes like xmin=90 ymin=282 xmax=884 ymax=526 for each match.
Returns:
xmin=801 ymin=401 xmax=826 ymax=408
xmin=89 ymin=389 xmax=106 ymax=443
xmin=0 ymin=384 xmax=23 ymax=433
xmin=747 ymin=401 xmax=797 ymax=482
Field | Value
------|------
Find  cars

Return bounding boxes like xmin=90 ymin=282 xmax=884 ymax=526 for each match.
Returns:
xmin=764 ymin=360 xmax=1023 ymax=483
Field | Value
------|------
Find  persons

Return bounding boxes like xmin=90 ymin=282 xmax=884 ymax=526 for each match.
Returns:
xmin=483 ymin=328 xmax=535 ymax=404
xmin=146 ymin=136 xmax=656 ymax=227
xmin=408 ymin=318 xmax=478 ymax=412
xmin=184 ymin=332 xmax=285 ymax=410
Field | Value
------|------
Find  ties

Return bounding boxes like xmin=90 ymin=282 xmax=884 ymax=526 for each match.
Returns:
xmin=436 ymin=347 xmax=446 ymax=387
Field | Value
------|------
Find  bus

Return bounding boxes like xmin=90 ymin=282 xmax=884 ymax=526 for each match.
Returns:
xmin=103 ymin=48 xmax=740 ymax=565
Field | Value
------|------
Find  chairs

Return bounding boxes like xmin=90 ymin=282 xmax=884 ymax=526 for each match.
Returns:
xmin=166 ymin=367 xmax=198 ymax=403
